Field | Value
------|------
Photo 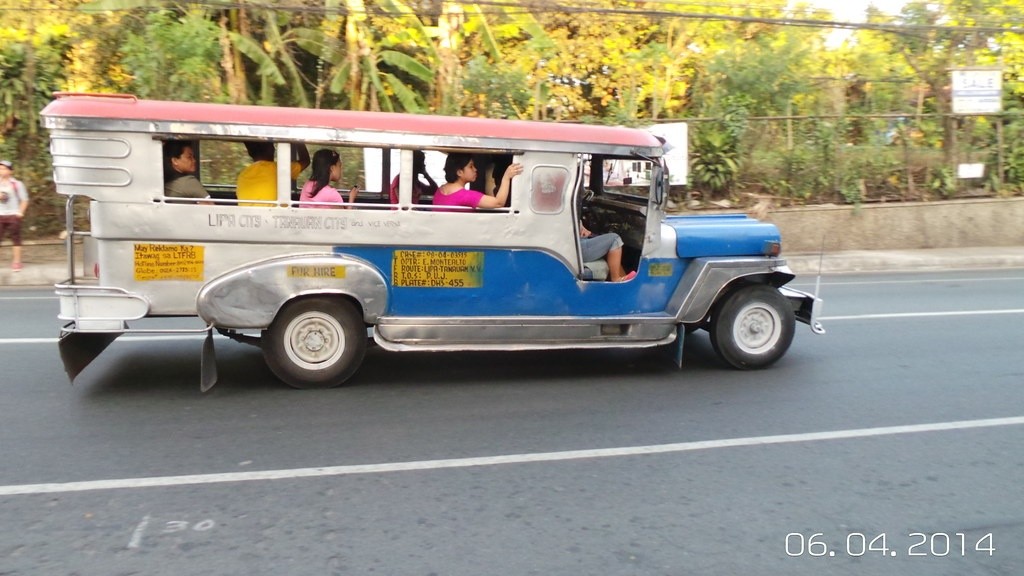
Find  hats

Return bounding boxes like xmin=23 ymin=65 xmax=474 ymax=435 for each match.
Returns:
xmin=0 ymin=160 xmax=13 ymax=167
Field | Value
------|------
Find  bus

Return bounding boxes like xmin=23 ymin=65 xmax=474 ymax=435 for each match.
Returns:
xmin=39 ymin=90 xmax=828 ymax=395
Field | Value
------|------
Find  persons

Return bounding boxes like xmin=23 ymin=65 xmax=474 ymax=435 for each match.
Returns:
xmin=163 ymin=140 xmax=215 ymax=205
xmin=579 ymin=221 xmax=636 ymax=282
xmin=299 ymin=149 xmax=359 ymax=209
xmin=432 ymin=152 xmax=523 ymax=212
xmin=492 ymin=159 xmax=511 ymax=207
xmin=0 ymin=161 xmax=29 ymax=271
xmin=236 ymin=142 xmax=310 ymax=208
xmin=390 ymin=150 xmax=438 ymax=210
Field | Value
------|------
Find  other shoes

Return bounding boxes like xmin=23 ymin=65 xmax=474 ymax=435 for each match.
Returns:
xmin=12 ymin=263 xmax=23 ymax=272
xmin=619 ymin=270 xmax=636 ymax=281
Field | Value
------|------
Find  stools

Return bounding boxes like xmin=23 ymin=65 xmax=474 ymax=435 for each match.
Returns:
xmin=582 ymin=258 xmax=609 ymax=281
